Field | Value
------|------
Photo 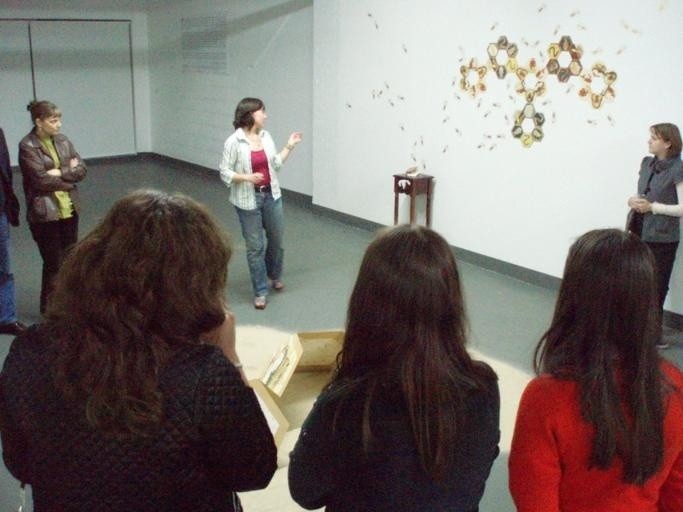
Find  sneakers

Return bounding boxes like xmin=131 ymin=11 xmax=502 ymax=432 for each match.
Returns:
xmin=272 ymin=277 xmax=284 ymax=289
xmin=253 ymin=296 xmax=266 ymax=309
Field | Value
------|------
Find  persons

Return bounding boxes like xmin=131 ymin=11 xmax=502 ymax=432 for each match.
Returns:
xmin=0 ymin=190 xmax=277 ymax=511
xmin=288 ymin=222 xmax=500 ymax=511
xmin=508 ymin=228 xmax=683 ymax=511
xmin=0 ymin=127 xmax=31 ymax=335
xmin=17 ymin=100 xmax=88 ymax=312
xmin=623 ymin=123 xmax=682 ymax=349
xmin=218 ymin=97 xmax=303 ymax=309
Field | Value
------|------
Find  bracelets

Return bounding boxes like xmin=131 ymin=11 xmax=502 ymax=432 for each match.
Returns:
xmin=284 ymin=143 xmax=294 ymax=151
xmin=234 ymin=362 xmax=242 ymax=368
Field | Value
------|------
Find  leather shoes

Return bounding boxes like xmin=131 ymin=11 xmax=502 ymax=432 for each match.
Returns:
xmin=1 ymin=319 xmax=28 ymax=336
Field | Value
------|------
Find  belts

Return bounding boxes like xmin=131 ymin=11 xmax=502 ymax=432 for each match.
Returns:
xmin=253 ymin=186 xmax=271 ymax=195
